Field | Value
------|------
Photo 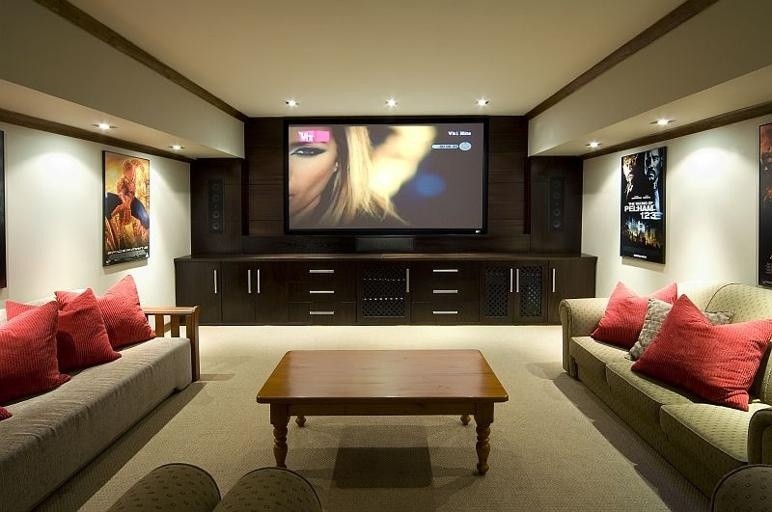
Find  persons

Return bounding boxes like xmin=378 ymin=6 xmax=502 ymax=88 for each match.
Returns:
xmin=622 ymin=154 xmax=642 ymax=201
xmin=286 ymin=124 xmax=408 ymax=229
xmin=643 ymin=149 xmax=664 ymax=214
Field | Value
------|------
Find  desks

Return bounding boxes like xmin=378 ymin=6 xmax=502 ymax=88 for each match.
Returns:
xmin=255 ymin=349 xmax=511 ymax=475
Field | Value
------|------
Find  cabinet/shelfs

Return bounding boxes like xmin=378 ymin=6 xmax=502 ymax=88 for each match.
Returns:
xmin=547 ymin=255 xmax=595 ymax=324
xmin=221 ymin=257 xmax=286 ymax=326
xmin=288 ymin=256 xmax=356 ymax=327
xmin=175 ymin=258 xmax=221 ymax=326
xmin=410 ymin=257 xmax=480 ymax=326
xmin=358 ymin=258 xmax=409 ymax=324
xmin=482 ymin=257 xmax=547 ymax=325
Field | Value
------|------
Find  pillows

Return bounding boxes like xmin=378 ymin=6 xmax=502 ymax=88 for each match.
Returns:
xmin=1 ymin=301 xmax=73 ymax=404
xmin=629 ymin=289 xmax=772 ymax=412
xmin=590 ymin=280 xmax=679 ymax=348
xmin=623 ymin=297 xmax=734 ymax=364
xmin=5 ymin=286 xmax=122 ymax=371
xmin=53 ymin=273 xmax=158 ymax=352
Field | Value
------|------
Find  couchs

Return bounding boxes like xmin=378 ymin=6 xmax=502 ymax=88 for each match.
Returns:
xmin=209 ymin=462 xmax=772 ymax=511
xmin=1 ymin=300 xmax=206 ymax=509
xmin=557 ymin=279 xmax=771 ymax=500
xmin=103 ymin=463 xmax=222 ymax=511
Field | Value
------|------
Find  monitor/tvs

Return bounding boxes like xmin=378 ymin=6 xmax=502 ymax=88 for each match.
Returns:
xmin=285 ymin=120 xmax=490 ymax=248
